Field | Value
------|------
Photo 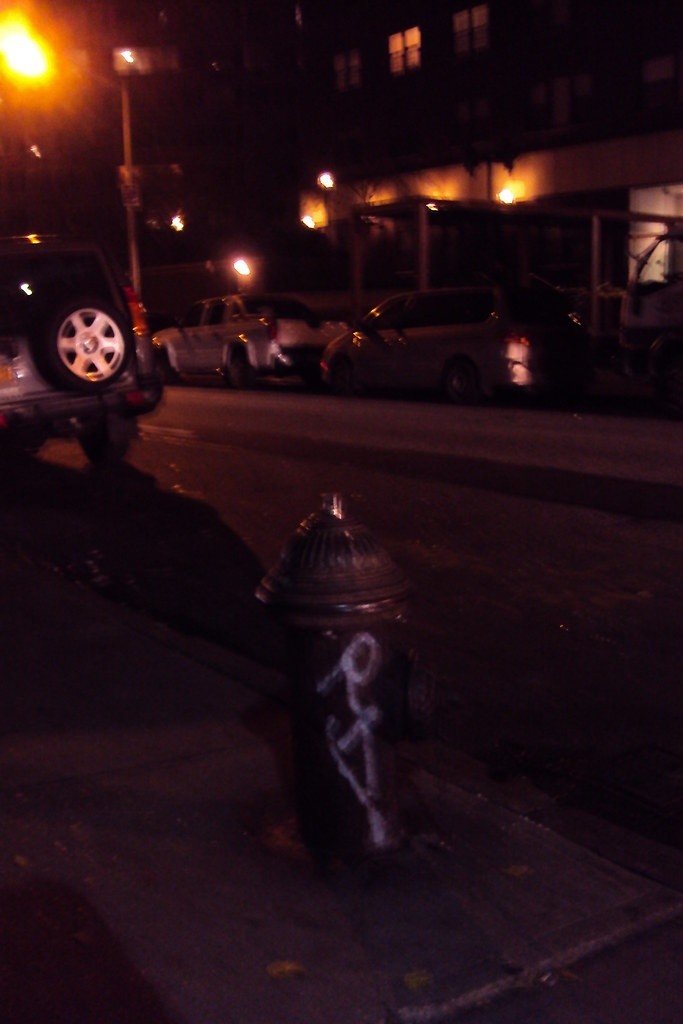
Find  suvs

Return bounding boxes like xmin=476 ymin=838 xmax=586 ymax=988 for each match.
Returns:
xmin=1 ymin=231 xmax=170 ymax=471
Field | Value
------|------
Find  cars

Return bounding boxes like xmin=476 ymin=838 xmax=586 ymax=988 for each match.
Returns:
xmin=319 ymin=285 xmax=553 ymax=408
xmin=148 ymin=290 xmax=331 ymax=392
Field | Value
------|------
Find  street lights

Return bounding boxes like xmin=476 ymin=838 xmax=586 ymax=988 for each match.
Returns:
xmin=104 ymin=45 xmax=155 ymax=301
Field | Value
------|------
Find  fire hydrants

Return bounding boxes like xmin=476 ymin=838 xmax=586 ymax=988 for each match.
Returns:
xmin=256 ymin=489 xmax=467 ymax=877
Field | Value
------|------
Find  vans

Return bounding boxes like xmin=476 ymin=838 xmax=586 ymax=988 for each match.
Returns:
xmin=617 ymin=220 xmax=683 ymax=414
